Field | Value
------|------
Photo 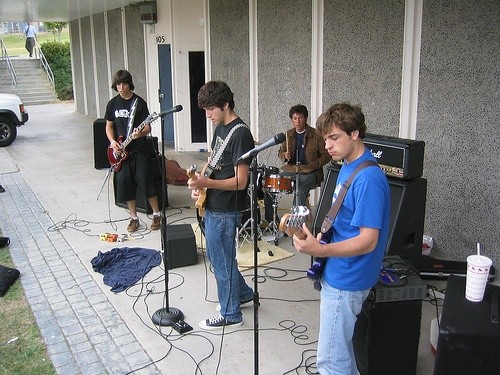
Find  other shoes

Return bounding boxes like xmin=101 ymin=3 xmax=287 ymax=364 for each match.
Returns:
xmin=150 ymin=215 xmax=162 ymax=230
xmin=127 ymin=217 xmax=139 ymax=232
xmin=0 ymin=236 xmax=10 ymax=248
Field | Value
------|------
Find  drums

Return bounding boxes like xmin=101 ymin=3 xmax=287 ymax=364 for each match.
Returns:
xmin=252 ymin=164 xmax=279 ymax=198
xmin=195 ymin=193 xmax=261 ymax=238
xmin=265 ymin=173 xmax=294 ymax=194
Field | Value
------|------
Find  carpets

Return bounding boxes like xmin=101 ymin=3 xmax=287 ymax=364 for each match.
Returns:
xmin=191 ymin=222 xmax=294 ymax=273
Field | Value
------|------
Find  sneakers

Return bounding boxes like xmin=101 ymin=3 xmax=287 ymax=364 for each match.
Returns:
xmin=199 ymin=315 xmax=245 ymax=331
xmin=216 ymin=299 xmax=254 ymax=313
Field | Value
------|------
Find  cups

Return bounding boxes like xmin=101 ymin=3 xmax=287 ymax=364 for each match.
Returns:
xmin=465 ymin=253 xmax=492 ymax=302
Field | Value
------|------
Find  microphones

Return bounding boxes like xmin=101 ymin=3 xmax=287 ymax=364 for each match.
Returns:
xmin=154 ymin=105 xmax=183 ymax=118
xmin=236 ymin=132 xmax=287 ymax=162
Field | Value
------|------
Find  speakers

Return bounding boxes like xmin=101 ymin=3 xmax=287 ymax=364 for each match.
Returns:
xmin=309 ymin=163 xmax=427 ymax=272
xmin=159 ymin=224 xmax=197 ymax=270
xmin=431 ymin=271 xmax=500 ymax=375
xmin=352 ymin=250 xmax=426 ymax=374
xmin=114 ymin=153 xmax=170 ymax=213
xmin=93 ymin=118 xmax=118 ymax=170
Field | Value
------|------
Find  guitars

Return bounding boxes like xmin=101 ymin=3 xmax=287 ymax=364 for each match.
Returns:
xmin=108 ymin=111 xmax=158 ymax=172
xmin=278 ymin=206 xmax=328 ymax=292
xmin=187 ymin=165 xmax=207 ymax=217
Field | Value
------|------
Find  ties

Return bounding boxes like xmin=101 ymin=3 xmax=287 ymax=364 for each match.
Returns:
xmin=26 ymin=26 xmax=30 ymax=36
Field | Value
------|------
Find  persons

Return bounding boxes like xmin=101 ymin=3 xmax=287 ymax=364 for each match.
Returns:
xmin=262 ymin=104 xmax=327 ymax=231
xmin=21 ymin=21 xmax=37 ymax=58
xmin=104 ymin=70 xmax=163 ymax=232
xmin=186 ymin=81 xmax=260 ymax=330
xmin=292 ymin=103 xmax=390 ymax=375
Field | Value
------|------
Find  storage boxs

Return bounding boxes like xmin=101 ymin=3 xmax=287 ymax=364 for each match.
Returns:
xmin=429 ymin=314 xmax=442 ymax=359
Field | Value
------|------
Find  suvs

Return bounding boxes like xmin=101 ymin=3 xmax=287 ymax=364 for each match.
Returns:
xmin=0 ymin=93 xmax=29 ymax=148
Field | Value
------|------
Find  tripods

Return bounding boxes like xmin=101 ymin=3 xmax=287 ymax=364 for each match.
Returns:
xmin=235 ymin=169 xmax=291 ymax=257
